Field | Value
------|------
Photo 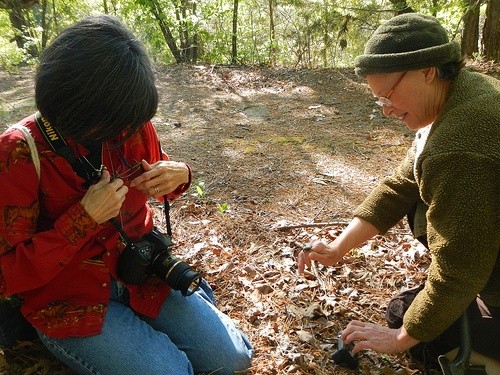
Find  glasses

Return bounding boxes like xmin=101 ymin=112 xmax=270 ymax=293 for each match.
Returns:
xmin=374 ymin=71 xmax=408 ymax=107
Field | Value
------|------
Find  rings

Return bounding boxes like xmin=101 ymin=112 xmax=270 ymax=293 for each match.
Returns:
xmin=154 ymin=186 xmax=160 ymax=195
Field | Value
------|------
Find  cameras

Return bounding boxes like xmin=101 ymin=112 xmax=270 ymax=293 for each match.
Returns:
xmin=116 ymin=228 xmax=201 ymax=297
xmin=331 ymin=332 xmax=358 ymax=369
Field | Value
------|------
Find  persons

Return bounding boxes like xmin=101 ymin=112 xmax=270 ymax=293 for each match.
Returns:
xmin=296 ymin=12 xmax=499 ymax=375
xmin=0 ymin=13 xmax=256 ymax=375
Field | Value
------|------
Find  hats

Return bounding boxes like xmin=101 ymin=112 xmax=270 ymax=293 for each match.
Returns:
xmin=353 ymin=13 xmax=462 ymax=77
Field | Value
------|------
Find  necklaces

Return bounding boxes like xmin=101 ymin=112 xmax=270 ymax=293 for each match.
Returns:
xmin=81 ymin=140 xmax=108 ymax=178
